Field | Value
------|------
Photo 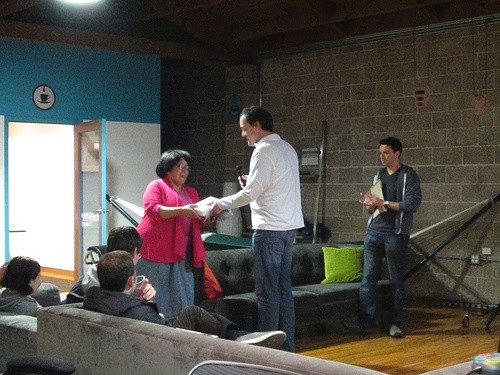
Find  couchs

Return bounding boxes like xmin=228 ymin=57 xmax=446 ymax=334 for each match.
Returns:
xmin=0 ymin=241 xmax=500 ymax=375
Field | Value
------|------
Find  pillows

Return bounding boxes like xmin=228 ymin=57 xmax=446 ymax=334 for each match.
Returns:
xmin=322 ymin=245 xmax=366 ymax=283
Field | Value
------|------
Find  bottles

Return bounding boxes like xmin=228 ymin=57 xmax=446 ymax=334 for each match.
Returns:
xmin=462 ymin=314 xmax=470 ymax=335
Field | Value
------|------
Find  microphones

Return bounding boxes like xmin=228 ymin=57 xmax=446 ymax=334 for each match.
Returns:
xmin=235 ymin=165 xmax=247 ymax=187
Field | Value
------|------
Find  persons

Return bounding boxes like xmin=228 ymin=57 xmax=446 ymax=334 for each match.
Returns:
xmin=340 ymin=137 xmax=422 ymax=338
xmin=83 ymin=250 xmax=287 ymax=349
xmin=0 ymin=255 xmax=43 ymax=317
xmin=135 ymin=149 xmax=216 ymax=319
xmin=66 ymin=225 xmax=144 ymax=304
xmin=211 ymin=105 xmax=305 ymax=353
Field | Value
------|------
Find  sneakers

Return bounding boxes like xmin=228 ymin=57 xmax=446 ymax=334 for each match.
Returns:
xmin=234 ymin=331 xmax=287 ymax=349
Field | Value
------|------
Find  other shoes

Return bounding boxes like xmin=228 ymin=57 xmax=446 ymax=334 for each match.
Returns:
xmin=389 ymin=326 xmax=402 ymax=338
xmin=341 ymin=320 xmax=375 ymax=334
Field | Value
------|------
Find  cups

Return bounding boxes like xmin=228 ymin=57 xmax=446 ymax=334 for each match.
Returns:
xmin=134 ymin=275 xmax=148 ymax=301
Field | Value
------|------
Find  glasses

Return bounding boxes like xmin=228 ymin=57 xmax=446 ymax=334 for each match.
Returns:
xmin=138 ymin=250 xmax=143 ymax=260
xmin=176 ymin=163 xmax=191 ymax=171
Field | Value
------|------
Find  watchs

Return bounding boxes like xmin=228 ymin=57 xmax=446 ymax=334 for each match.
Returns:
xmin=384 ymin=200 xmax=390 ymax=209
